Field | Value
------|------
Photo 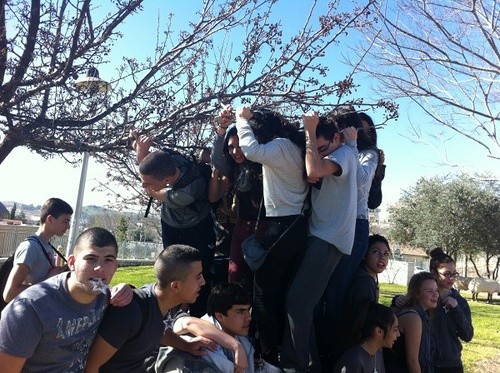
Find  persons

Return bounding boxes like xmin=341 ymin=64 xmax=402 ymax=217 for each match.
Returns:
xmin=282 ymin=109 xmax=358 ymax=373
xmin=156 ymin=282 xmax=281 ymax=373
xmin=2 ymin=198 xmax=74 ymax=303
xmin=174 ymin=312 xmax=248 ymax=372
xmin=135 ymin=134 xmax=217 ymax=312
xmin=211 ymin=99 xmax=386 ymax=372
xmin=85 ymin=245 xmax=218 ymax=373
xmin=352 ymin=232 xmax=391 ymax=312
xmin=333 ymin=301 xmax=399 ymax=373
xmin=390 ymin=271 xmax=439 ymax=373
xmin=392 ymin=247 xmax=473 ymax=373
xmin=0 ymin=227 xmax=133 ymax=372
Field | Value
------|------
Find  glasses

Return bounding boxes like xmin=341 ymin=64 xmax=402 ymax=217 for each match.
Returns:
xmin=440 ymin=271 xmax=459 ymax=279
xmin=318 ymin=141 xmax=331 ymax=152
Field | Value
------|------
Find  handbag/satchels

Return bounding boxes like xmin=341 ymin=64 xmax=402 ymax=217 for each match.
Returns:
xmin=241 ymin=235 xmax=267 ymax=271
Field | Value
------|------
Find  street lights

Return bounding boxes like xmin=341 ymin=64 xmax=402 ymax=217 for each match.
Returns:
xmin=61 ymin=63 xmax=112 ymax=275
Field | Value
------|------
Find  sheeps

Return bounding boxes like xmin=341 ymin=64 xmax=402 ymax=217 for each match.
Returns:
xmin=468 ymin=278 xmax=500 ymax=304
xmin=453 ymin=276 xmax=474 ymax=300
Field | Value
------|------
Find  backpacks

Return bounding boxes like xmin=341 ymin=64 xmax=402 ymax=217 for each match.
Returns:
xmin=168 ymin=150 xmax=223 ymax=213
xmin=0 ymin=235 xmax=67 ymax=309
